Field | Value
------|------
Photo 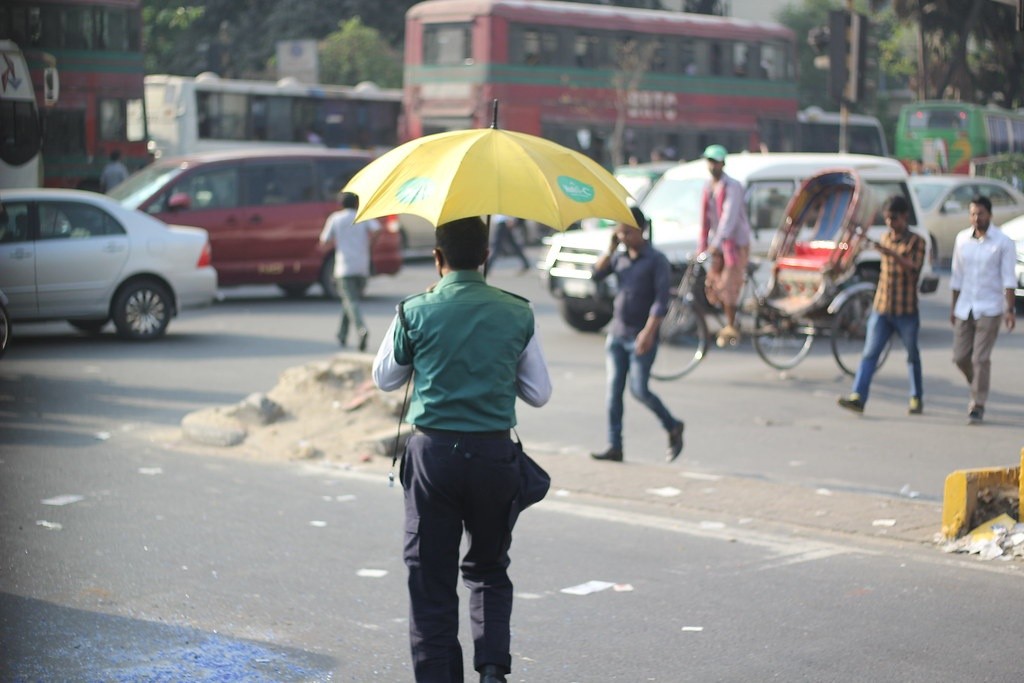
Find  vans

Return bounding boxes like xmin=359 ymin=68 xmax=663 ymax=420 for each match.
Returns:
xmin=800 ymin=110 xmax=889 ymax=158
xmin=98 ymin=151 xmax=405 ymax=301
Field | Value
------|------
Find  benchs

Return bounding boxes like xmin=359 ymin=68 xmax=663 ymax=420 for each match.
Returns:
xmin=761 ymin=240 xmax=856 ymax=319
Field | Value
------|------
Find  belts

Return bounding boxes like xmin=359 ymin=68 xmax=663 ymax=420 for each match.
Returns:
xmin=414 ymin=425 xmax=510 ymax=440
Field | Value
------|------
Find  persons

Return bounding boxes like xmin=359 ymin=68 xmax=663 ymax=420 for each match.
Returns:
xmin=100 ymin=149 xmax=129 ymax=193
xmin=317 ymin=191 xmax=379 ymax=350
xmin=837 ymin=195 xmax=925 ymax=414
xmin=590 ymin=207 xmax=683 ymax=464
xmin=372 ymin=215 xmax=552 ymax=683
xmin=485 ymin=215 xmax=530 ymax=273
xmin=951 ymin=196 xmax=1016 ymax=421
xmin=695 ymin=144 xmax=753 ymax=346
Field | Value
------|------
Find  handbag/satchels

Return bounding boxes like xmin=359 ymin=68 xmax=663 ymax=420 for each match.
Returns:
xmin=515 ymin=442 xmax=550 ymax=512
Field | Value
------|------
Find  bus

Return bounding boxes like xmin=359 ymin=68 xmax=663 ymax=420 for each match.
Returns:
xmin=897 ymin=103 xmax=1024 ymax=177
xmin=398 ymin=2 xmax=799 ymax=172
xmin=46 ymin=70 xmax=146 ymax=194
xmin=0 ymin=41 xmax=60 ymax=190
xmin=142 ymin=73 xmax=402 ymax=172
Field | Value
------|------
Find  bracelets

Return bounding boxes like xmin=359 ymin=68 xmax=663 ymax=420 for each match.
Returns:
xmin=605 ymin=249 xmax=613 ymax=257
xmin=1007 ymin=307 xmax=1017 ymax=315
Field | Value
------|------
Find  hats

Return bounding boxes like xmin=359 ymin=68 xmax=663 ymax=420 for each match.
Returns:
xmin=704 ymin=144 xmax=726 ymax=162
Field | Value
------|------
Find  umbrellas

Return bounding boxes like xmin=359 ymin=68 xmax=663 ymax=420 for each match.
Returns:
xmin=339 ymin=99 xmax=641 ymax=279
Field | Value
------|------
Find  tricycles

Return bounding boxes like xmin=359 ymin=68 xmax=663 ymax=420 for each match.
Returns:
xmin=652 ymin=168 xmax=895 ymax=381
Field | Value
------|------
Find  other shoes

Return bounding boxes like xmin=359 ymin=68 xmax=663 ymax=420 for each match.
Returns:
xmin=360 ymin=332 xmax=368 ymax=351
xmin=838 ymin=393 xmax=863 ymax=415
xmin=592 ymin=447 xmax=623 ymax=461
xmin=908 ymin=397 xmax=922 ymax=413
xmin=967 ymin=404 xmax=984 ymax=423
xmin=337 ymin=333 xmax=344 ymax=344
xmin=716 ymin=327 xmax=739 ymax=347
xmin=480 ymin=674 xmax=507 ymax=683
xmin=664 ymin=422 xmax=683 ymax=462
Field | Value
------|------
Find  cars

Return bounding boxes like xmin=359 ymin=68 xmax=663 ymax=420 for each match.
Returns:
xmin=915 ymin=175 xmax=1024 ymax=266
xmin=995 ymin=217 xmax=1024 ymax=301
xmin=0 ymin=189 xmax=218 ymax=342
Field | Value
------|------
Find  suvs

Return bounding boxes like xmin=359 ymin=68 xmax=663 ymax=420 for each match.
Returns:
xmin=535 ymin=149 xmax=932 ymax=337
